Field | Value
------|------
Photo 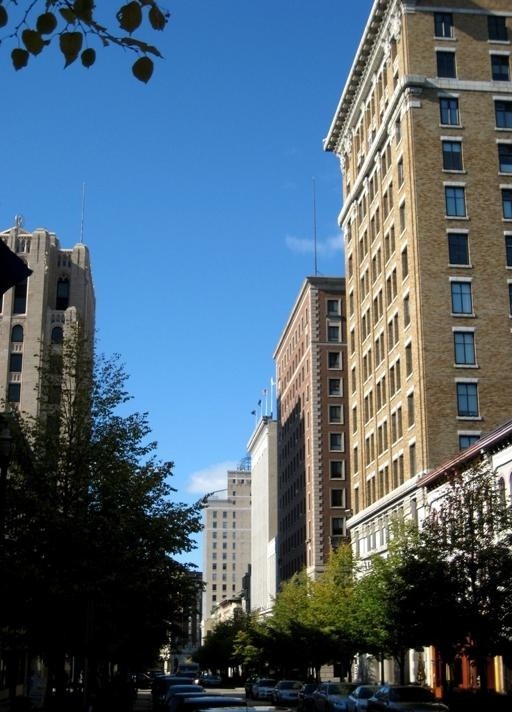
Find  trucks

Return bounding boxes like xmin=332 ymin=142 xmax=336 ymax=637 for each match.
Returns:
xmin=169 ymin=654 xmax=200 ymax=677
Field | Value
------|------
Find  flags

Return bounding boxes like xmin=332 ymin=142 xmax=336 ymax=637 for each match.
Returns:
xmin=261 ymin=387 xmax=267 ymax=396
xmin=250 ymin=410 xmax=256 ymax=416
xmin=257 ymin=399 xmax=261 ymax=407
xmin=270 ymin=376 xmax=276 ymax=386
